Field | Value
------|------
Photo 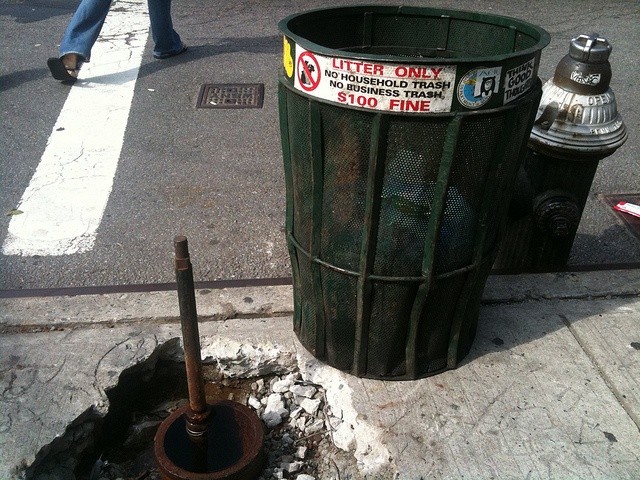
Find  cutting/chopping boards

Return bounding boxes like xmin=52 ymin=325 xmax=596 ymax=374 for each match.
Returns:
xmin=492 ymin=32 xmax=630 ymax=276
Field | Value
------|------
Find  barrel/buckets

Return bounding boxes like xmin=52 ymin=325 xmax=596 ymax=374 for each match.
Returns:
xmin=47 ymin=58 xmax=78 ymax=81
xmin=153 ymin=44 xmax=187 ymax=59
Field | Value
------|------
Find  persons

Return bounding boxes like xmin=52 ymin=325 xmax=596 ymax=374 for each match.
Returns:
xmin=47 ymin=0 xmax=186 ymax=81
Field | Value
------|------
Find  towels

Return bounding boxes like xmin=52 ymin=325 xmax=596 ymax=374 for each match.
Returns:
xmin=278 ymin=4 xmax=553 ymax=381
